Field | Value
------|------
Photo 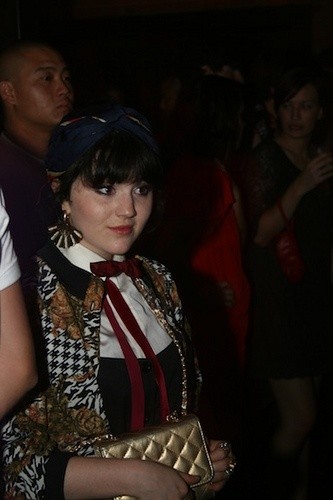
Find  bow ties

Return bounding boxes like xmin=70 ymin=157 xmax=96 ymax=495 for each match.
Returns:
xmin=90 ymin=259 xmax=142 ymax=279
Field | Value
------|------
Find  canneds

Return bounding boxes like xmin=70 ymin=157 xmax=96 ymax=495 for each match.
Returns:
xmin=309 ymin=144 xmax=327 ymax=160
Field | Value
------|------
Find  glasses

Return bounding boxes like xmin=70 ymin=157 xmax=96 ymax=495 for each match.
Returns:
xmin=46 ymin=106 xmax=153 ymax=172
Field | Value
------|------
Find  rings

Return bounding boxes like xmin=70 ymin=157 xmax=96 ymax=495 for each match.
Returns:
xmin=225 ymin=469 xmax=233 ymax=480
xmin=216 ymin=440 xmax=232 ymax=452
xmin=228 ymin=455 xmax=236 ymax=471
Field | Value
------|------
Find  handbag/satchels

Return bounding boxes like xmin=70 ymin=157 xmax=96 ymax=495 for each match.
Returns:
xmin=94 ymin=414 xmax=213 ymax=500
xmin=274 ymin=198 xmax=304 ymax=283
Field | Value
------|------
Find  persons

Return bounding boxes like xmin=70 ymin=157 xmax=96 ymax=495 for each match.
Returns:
xmin=0 ymin=40 xmax=333 ymax=500
xmin=0 ymin=107 xmax=237 ymax=500
